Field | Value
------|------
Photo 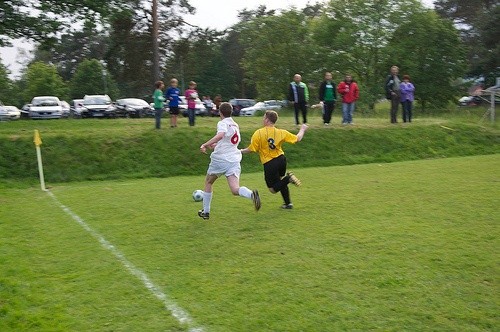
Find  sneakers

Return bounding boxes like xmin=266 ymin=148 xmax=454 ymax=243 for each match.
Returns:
xmin=251 ymin=189 xmax=261 ymax=211
xmin=198 ymin=209 xmax=210 ymax=221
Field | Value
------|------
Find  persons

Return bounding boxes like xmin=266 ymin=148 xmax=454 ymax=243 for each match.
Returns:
xmin=198 ymin=102 xmax=261 ymax=219
xmin=203 ymin=94 xmax=222 ymax=112
xmin=165 ymin=78 xmax=183 ymax=128
xmin=288 ymin=73 xmax=309 ymax=127
xmin=239 ymin=110 xmax=309 ymax=208
xmin=184 ymin=82 xmax=198 ymax=126
xmin=399 ymin=75 xmax=414 ymax=122
xmin=337 ymin=74 xmax=359 ymax=125
xmin=152 ymin=81 xmax=170 ymax=129
xmin=319 ymin=71 xmax=337 ymax=126
xmin=385 ymin=65 xmax=401 ymax=123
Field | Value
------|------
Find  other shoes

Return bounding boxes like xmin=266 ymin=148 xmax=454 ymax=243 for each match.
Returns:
xmin=287 ymin=171 xmax=302 ymax=188
xmin=282 ymin=203 xmax=293 ymax=209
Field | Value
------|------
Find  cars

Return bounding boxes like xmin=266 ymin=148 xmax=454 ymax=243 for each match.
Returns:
xmin=20 ymin=93 xmax=152 ymax=121
xmin=239 ymin=101 xmax=281 ymax=118
xmin=0 ymin=102 xmax=21 ymax=121
xmin=457 ymin=94 xmax=484 ymax=106
xmin=177 ymin=95 xmax=206 ymax=116
xmin=229 ymin=98 xmax=255 ymax=116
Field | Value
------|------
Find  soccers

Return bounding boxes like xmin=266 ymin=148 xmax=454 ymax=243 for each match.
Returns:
xmin=192 ymin=189 xmax=203 ymax=202
